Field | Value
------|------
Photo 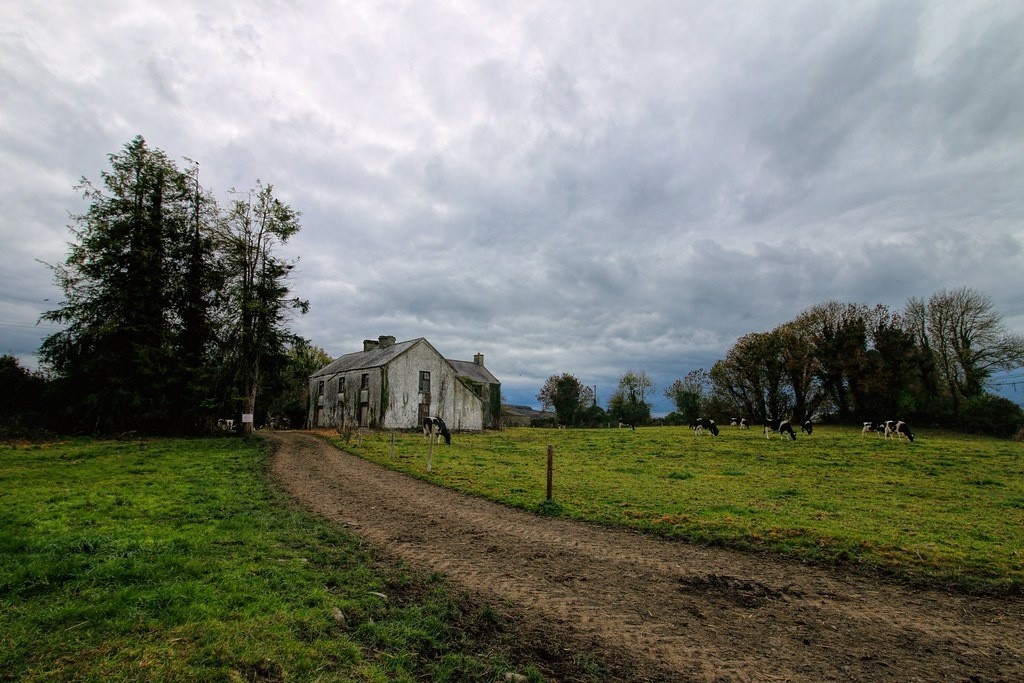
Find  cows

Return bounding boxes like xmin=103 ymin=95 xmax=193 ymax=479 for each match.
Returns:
xmin=729 ymin=417 xmax=750 ymax=430
xmin=694 ymin=418 xmax=721 ymax=437
xmin=422 ymin=415 xmax=451 ymax=446
xmin=883 ymin=420 xmax=916 ymax=443
xmin=800 ymin=417 xmax=813 ymax=435
xmin=763 ymin=418 xmax=797 ymax=441
xmin=862 ymin=421 xmax=885 ymax=437
xmin=558 ymin=422 xmax=567 ymax=429
xmin=617 ymin=417 xmax=636 ymax=431
